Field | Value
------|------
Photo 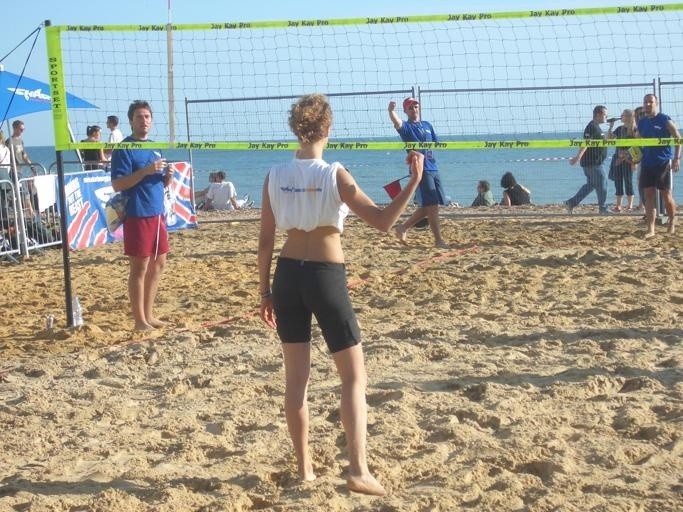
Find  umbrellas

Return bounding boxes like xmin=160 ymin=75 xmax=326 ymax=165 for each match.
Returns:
xmin=0 ymin=63 xmax=101 ymax=134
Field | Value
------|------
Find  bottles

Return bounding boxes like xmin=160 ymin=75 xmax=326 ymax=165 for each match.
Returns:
xmin=606 ymin=117 xmax=621 ymax=123
xmin=73 ymin=297 xmax=84 ymax=329
xmin=46 ymin=315 xmax=55 ymax=329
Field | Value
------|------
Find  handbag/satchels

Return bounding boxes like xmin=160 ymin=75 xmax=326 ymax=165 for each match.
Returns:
xmin=608 ymin=154 xmax=622 ymax=181
xmin=106 ymin=194 xmax=128 ymax=233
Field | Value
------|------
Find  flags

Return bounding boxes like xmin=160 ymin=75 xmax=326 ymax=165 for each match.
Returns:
xmin=384 ymin=179 xmax=403 ymax=199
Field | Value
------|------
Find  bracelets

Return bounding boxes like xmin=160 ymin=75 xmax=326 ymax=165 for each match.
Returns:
xmin=259 ymin=289 xmax=272 ymax=298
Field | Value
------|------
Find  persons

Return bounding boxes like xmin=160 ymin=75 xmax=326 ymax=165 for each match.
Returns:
xmin=111 ymin=100 xmax=174 ymax=331
xmin=0 ymin=128 xmax=12 ymax=175
xmin=194 ymin=172 xmax=216 ymax=197
xmin=470 ymin=181 xmax=494 ymax=206
xmin=258 ymin=93 xmax=423 ymax=496
xmin=498 ymin=172 xmax=531 ymax=207
xmin=203 ymin=172 xmax=249 ymax=211
xmin=107 ymin=115 xmax=124 ymax=144
xmin=5 ymin=121 xmax=37 ymax=218
xmin=78 ymin=125 xmax=107 ymax=170
xmin=562 ymin=94 xmax=681 ymax=240
xmin=387 ymin=97 xmax=449 ymax=249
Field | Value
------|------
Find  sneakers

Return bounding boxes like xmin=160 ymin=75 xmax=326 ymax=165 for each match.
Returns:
xmin=243 ymin=194 xmax=249 ymax=202
xmin=563 ymin=201 xmax=572 ymax=216
xmin=246 ymin=201 xmax=254 ymax=209
xmin=599 ymin=210 xmax=614 ymax=216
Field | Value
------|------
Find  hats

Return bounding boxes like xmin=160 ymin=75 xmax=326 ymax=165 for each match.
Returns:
xmin=403 ymin=98 xmax=419 ymax=111
